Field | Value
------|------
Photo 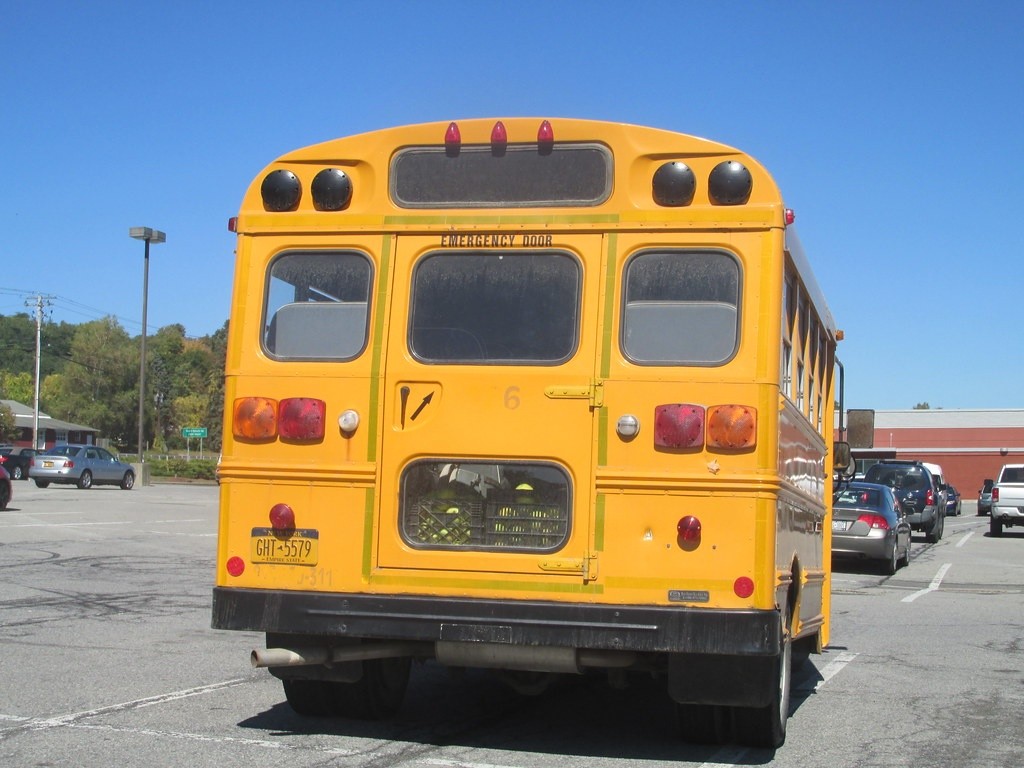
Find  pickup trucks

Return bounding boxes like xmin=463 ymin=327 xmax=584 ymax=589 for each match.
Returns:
xmin=988 ymin=463 xmax=1024 ymax=537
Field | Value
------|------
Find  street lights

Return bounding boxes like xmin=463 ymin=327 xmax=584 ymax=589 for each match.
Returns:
xmin=129 ymin=226 xmax=167 ymax=463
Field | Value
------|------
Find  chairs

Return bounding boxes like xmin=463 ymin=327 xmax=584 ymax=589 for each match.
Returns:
xmin=264 ymin=302 xmax=366 ymax=356
xmin=624 ymin=299 xmax=738 ymax=363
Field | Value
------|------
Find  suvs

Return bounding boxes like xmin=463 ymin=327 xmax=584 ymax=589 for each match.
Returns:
xmin=863 ymin=458 xmax=948 ymax=543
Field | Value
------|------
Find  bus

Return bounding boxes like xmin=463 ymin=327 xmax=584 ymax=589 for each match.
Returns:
xmin=209 ymin=115 xmax=852 ymax=753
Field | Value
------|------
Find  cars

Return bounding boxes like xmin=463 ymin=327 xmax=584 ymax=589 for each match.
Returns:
xmin=977 ymin=482 xmax=996 ymax=516
xmin=831 ymin=480 xmax=916 ymax=575
xmin=28 ymin=443 xmax=137 ymax=490
xmin=0 ymin=444 xmax=48 ymax=511
xmin=945 ymin=486 xmax=962 ymax=517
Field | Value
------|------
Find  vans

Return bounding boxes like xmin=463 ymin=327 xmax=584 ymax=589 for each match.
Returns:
xmin=910 ymin=462 xmax=950 ymax=517
